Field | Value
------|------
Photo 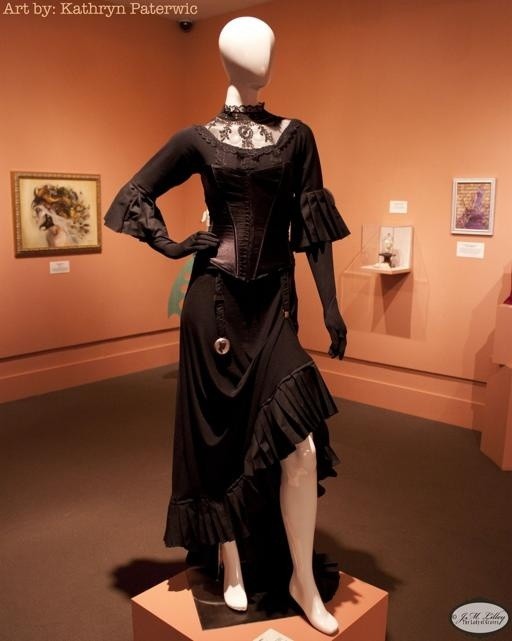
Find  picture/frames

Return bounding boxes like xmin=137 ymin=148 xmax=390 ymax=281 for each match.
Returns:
xmin=450 ymin=178 xmax=496 ymax=236
xmin=11 ymin=171 xmax=101 ymax=257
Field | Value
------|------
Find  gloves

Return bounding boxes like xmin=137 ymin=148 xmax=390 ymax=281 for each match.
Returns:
xmin=146 ymin=230 xmax=221 ymax=260
xmin=305 ymin=241 xmax=348 ymax=360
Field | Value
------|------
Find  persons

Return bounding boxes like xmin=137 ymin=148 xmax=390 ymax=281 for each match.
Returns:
xmin=102 ymin=15 xmax=354 ymax=640
